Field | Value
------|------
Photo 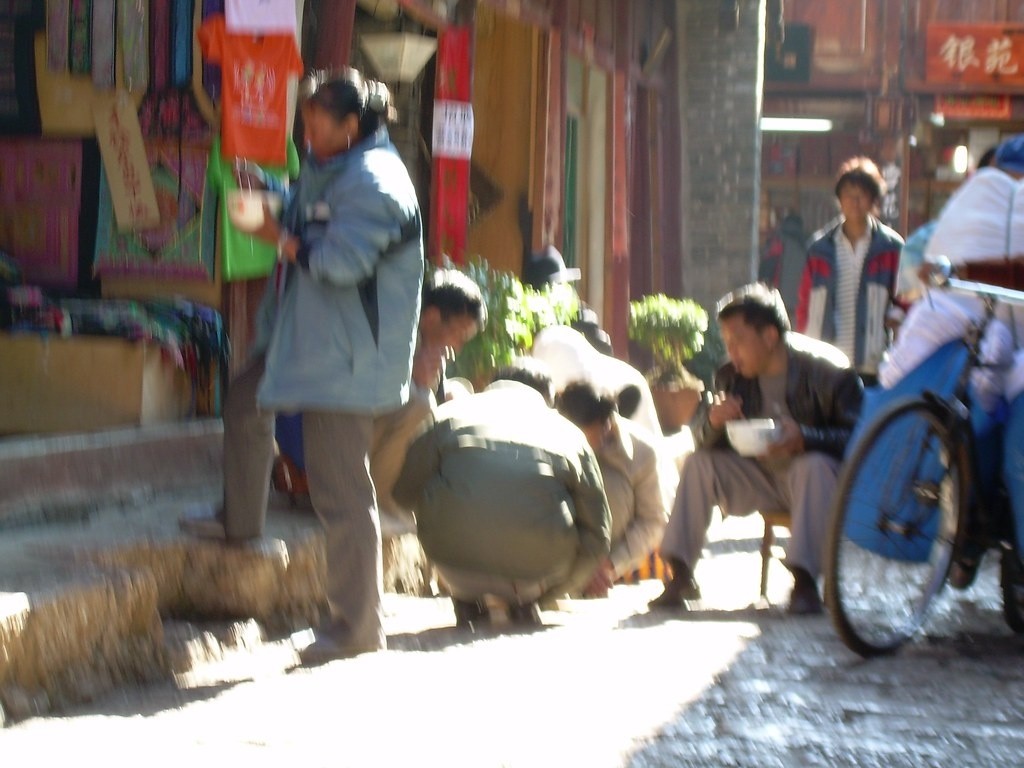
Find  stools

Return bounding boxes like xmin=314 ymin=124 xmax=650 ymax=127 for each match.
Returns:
xmin=757 ymin=510 xmax=829 ymax=606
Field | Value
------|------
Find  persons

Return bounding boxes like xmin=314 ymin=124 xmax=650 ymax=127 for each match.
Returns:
xmin=229 ymin=67 xmax=425 ymax=667
xmin=275 ymin=136 xmax=1022 ymax=645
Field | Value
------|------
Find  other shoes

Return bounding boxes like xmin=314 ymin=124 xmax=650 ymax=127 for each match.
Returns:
xmin=179 ymin=515 xmax=225 ymax=539
xmin=301 ymin=637 xmax=357 ymax=662
xmin=648 ymin=569 xmax=701 ymax=608
xmin=791 ymin=571 xmax=821 ymax=612
xmin=950 ymin=554 xmax=986 ymax=589
xmin=451 ymin=602 xmax=543 ymax=627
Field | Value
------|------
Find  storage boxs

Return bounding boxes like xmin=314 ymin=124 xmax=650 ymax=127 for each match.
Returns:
xmin=0 ymin=334 xmax=191 ymax=438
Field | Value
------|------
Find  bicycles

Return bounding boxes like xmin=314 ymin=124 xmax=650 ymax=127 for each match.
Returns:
xmin=822 ymin=254 xmax=1024 ymax=663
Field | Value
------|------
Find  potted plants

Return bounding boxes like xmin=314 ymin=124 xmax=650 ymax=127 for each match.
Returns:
xmin=628 ymin=291 xmax=710 ymax=434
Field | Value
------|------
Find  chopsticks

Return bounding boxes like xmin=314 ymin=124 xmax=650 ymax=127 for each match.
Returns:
xmin=715 ymin=390 xmax=747 ymax=422
xmin=235 ymin=153 xmax=252 ymax=198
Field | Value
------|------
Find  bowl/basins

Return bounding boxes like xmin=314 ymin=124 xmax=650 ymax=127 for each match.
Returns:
xmin=227 ymin=191 xmax=282 ymax=234
xmin=726 ymin=417 xmax=783 ymax=457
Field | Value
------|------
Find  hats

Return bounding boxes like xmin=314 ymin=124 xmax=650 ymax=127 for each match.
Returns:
xmin=996 ymin=134 xmax=1024 ymax=173
xmin=527 ymin=245 xmax=582 ymax=292
